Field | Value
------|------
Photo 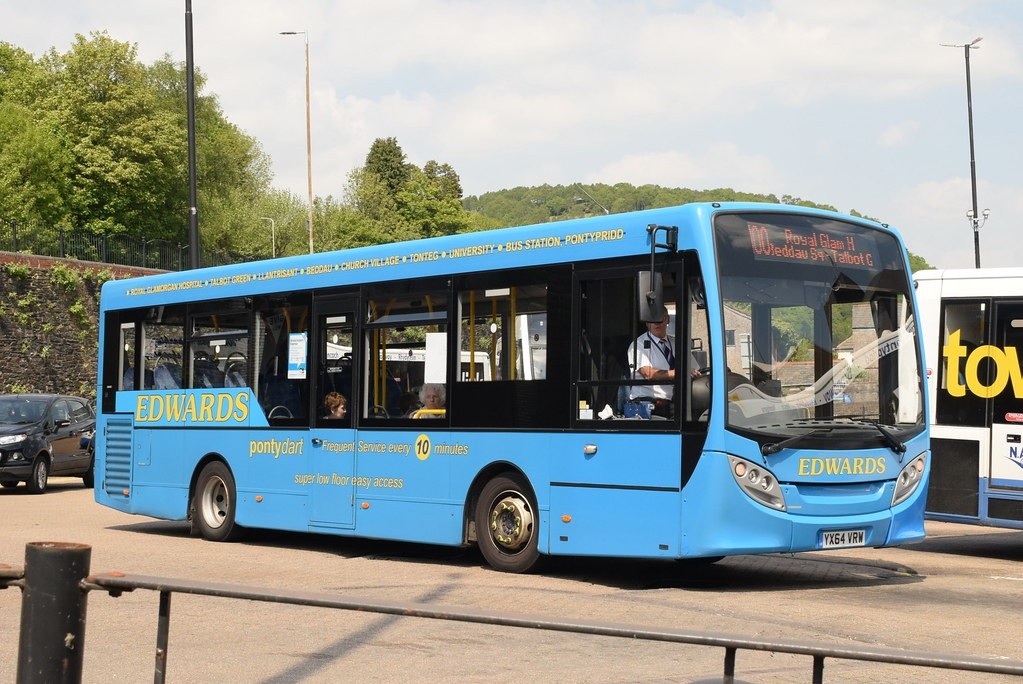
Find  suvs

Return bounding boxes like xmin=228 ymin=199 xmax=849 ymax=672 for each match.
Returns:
xmin=0 ymin=393 xmax=96 ymax=493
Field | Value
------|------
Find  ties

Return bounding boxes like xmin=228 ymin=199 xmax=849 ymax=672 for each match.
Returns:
xmin=659 ymin=339 xmax=675 ymax=370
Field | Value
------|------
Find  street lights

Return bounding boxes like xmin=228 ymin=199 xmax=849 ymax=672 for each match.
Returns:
xmin=280 ymin=30 xmax=313 ymax=252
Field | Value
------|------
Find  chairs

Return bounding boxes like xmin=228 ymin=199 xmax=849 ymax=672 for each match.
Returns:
xmin=123 ymin=351 xmax=406 ymax=421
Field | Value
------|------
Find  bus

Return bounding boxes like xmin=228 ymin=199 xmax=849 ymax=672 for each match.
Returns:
xmin=897 ymin=266 xmax=1023 ymax=530
xmin=93 ymin=202 xmax=930 ymax=574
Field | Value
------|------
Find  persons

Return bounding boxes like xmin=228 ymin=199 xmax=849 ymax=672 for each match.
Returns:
xmin=321 ymin=392 xmax=347 ymax=420
xmin=409 ymin=383 xmax=447 ymax=420
xmin=628 ymin=306 xmax=704 ymax=419
